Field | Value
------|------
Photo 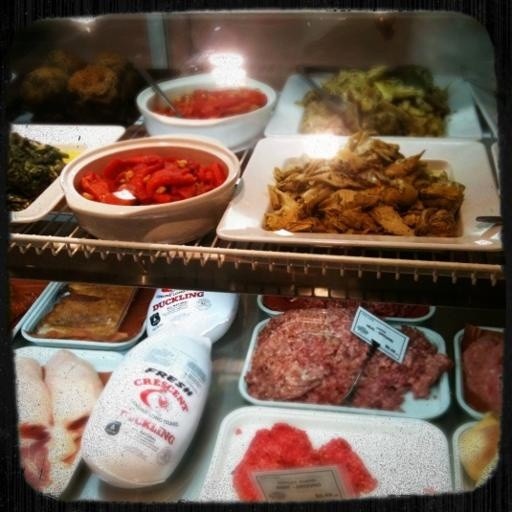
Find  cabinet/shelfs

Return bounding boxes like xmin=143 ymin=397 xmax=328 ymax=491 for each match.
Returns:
xmin=4 ymin=10 xmax=502 ymax=501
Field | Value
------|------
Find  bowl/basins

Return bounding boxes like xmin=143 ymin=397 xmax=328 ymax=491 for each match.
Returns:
xmin=59 ymin=134 xmax=244 ymax=244
xmin=136 ymin=71 xmax=277 ymax=154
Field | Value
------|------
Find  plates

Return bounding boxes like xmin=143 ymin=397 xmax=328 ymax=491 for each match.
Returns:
xmin=263 ymin=68 xmax=486 ymax=139
xmin=454 ymin=323 xmax=504 ymax=415
xmin=237 ymin=316 xmax=451 ymax=420
xmin=17 ymin=282 xmax=159 ymax=349
xmin=7 ymin=120 xmax=126 ymax=225
xmin=195 ymin=403 xmax=457 ymax=503
xmin=216 ymin=137 xmax=508 ymax=254
xmin=451 ymin=420 xmax=502 ymax=494
xmin=14 ymin=341 xmax=124 ymax=501
xmin=253 ymin=291 xmax=438 ymax=324
